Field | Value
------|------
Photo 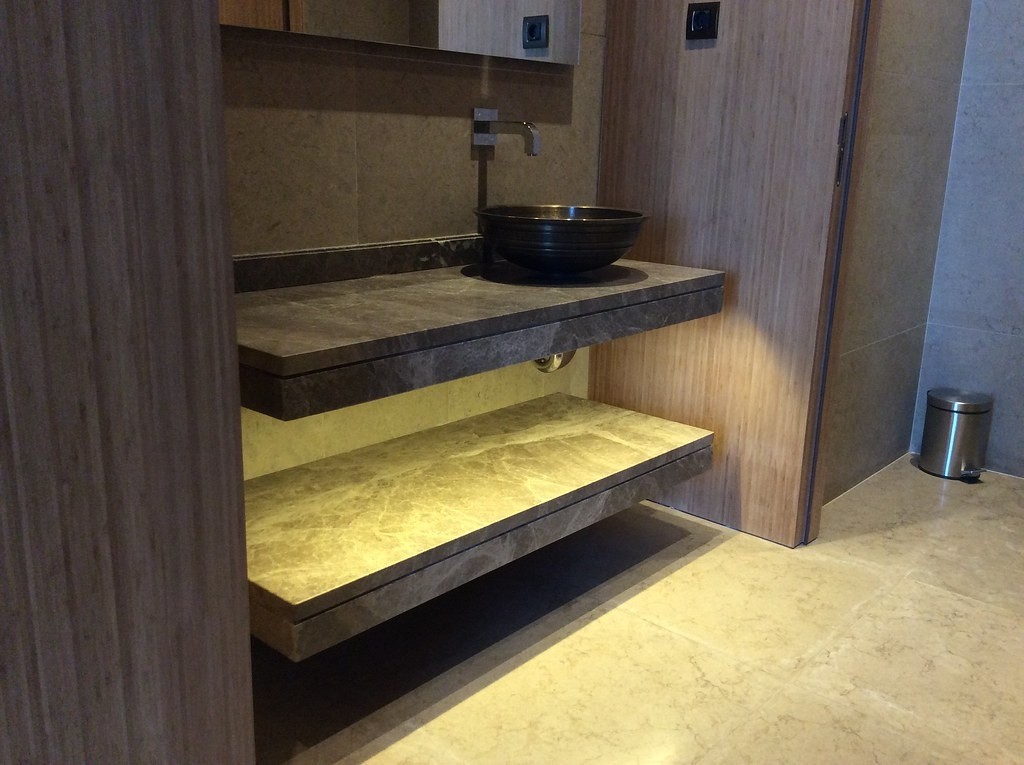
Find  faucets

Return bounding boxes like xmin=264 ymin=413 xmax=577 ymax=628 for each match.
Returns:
xmin=477 ymin=119 xmax=542 ymax=157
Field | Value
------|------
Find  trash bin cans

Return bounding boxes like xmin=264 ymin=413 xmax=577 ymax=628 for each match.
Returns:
xmin=917 ymin=385 xmax=995 ymax=481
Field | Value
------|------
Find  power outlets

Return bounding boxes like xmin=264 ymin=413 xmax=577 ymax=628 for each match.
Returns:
xmin=522 ymin=15 xmax=549 ymax=49
xmin=686 ymin=2 xmax=720 ymax=40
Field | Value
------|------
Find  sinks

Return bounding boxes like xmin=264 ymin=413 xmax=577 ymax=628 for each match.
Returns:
xmin=477 ymin=205 xmax=652 ymax=282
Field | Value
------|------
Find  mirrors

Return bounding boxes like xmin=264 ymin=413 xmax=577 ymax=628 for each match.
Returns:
xmin=218 ymin=0 xmax=582 ymax=65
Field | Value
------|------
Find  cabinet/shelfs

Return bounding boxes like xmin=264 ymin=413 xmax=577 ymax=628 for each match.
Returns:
xmin=231 ymin=257 xmax=724 ymax=662
